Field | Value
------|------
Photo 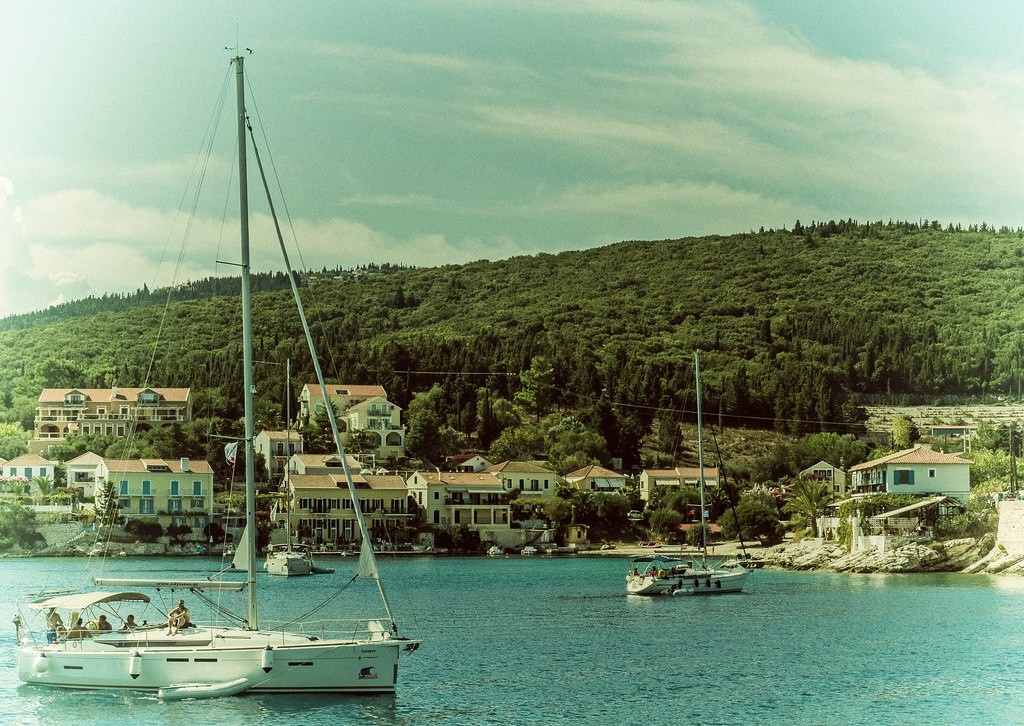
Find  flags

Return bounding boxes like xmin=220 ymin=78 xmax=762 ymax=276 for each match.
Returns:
xmin=225 ymin=441 xmax=236 ymax=462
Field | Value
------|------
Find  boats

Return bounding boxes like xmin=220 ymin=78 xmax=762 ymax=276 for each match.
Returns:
xmin=520 ymin=547 xmax=538 ymax=556
xmin=624 ymin=348 xmax=754 ymax=598
xmin=262 ymin=355 xmax=316 ymax=579
xmin=641 ymin=540 xmax=662 ymax=548
xmin=486 ymin=545 xmax=504 ymax=555
xmin=12 ymin=48 xmax=416 ymax=707
xmin=722 ymin=553 xmax=771 ymax=570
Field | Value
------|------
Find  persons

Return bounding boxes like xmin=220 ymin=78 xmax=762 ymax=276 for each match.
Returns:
xmin=121 ymin=615 xmax=138 ymax=630
xmin=46 ymin=607 xmax=63 ymax=644
xmin=97 ymin=615 xmax=112 ymax=630
xmin=650 ymin=566 xmax=656 ymax=577
xmin=66 ymin=618 xmax=93 ymax=640
xmin=166 ymin=599 xmax=190 ymax=637
